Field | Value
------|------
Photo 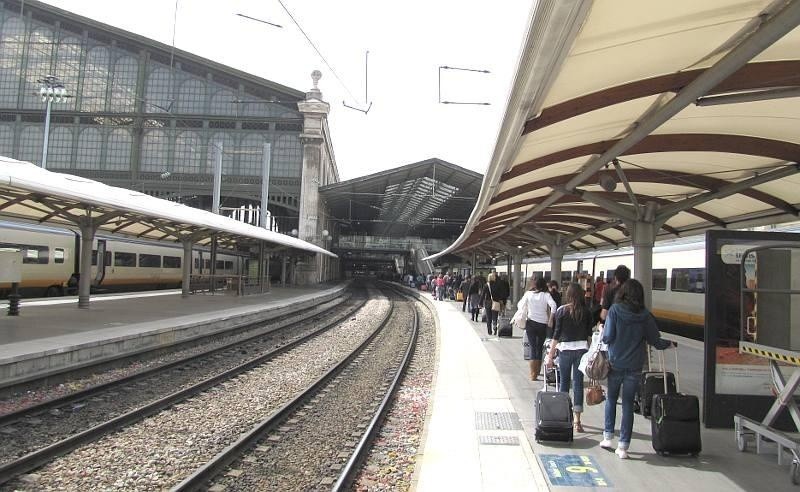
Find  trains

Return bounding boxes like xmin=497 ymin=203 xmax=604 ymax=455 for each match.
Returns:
xmin=491 ymin=223 xmax=756 ymax=340
xmin=0 ymin=218 xmax=280 ymax=298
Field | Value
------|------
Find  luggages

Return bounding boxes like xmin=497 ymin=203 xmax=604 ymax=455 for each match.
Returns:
xmin=535 ymin=365 xmax=573 ymax=445
xmin=651 ymin=342 xmax=701 ymax=457
xmin=640 ymin=344 xmax=676 ymax=418
xmin=498 ymin=305 xmax=512 ymax=337
xmin=482 ymin=307 xmax=487 ymax=321
xmin=524 ymin=330 xmax=530 ymax=360
xmin=545 ymin=357 xmax=560 ymax=386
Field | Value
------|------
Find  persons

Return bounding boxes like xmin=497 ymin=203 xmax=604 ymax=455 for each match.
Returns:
xmin=398 ymin=268 xmax=464 ymax=302
xmin=545 ymin=282 xmax=593 ymax=433
xmin=599 ymin=279 xmax=677 ymax=459
xmin=600 ymin=265 xmax=632 ymax=321
xmin=477 ymin=275 xmax=504 ymax=335
xmin=516 ymin=275 xmax=557 ymax=382
xmin=457 ymin=268 xmax=562 ymax=328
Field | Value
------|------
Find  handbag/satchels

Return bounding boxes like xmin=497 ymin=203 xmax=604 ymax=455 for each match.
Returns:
xmin=577 ymin=332 xmax=609 ymax=376
xmin=585 ymin=351 xmax=610 ymax=380
xmin=510 ymin=298 xmax=527 ymax=329
xmin=456 ymin=290 xmax=464 ymax=300
xmin=491 ymin=301 xmax=500 ymax=312
xmin=586 ymin=384 xmax=606 ymax=405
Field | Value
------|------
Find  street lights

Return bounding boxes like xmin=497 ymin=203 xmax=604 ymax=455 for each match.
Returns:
xmin=36 ymin=75 xmax=73 ymax=169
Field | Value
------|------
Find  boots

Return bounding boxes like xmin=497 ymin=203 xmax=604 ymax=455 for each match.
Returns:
xmin=529 ymin=360 xmax=541 ymax=381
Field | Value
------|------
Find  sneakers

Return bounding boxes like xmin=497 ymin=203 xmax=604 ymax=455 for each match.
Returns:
xmin=633 ymin=399 xmax=640 ymax=414
xmin=600 ymin=440 xmax=613 ymax=448
xmin=615 ymin=448 xmax=628 ymax=458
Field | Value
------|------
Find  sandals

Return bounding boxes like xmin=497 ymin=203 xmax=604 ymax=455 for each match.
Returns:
xmin=574 ymin=421 xmax=584 ymax=433
